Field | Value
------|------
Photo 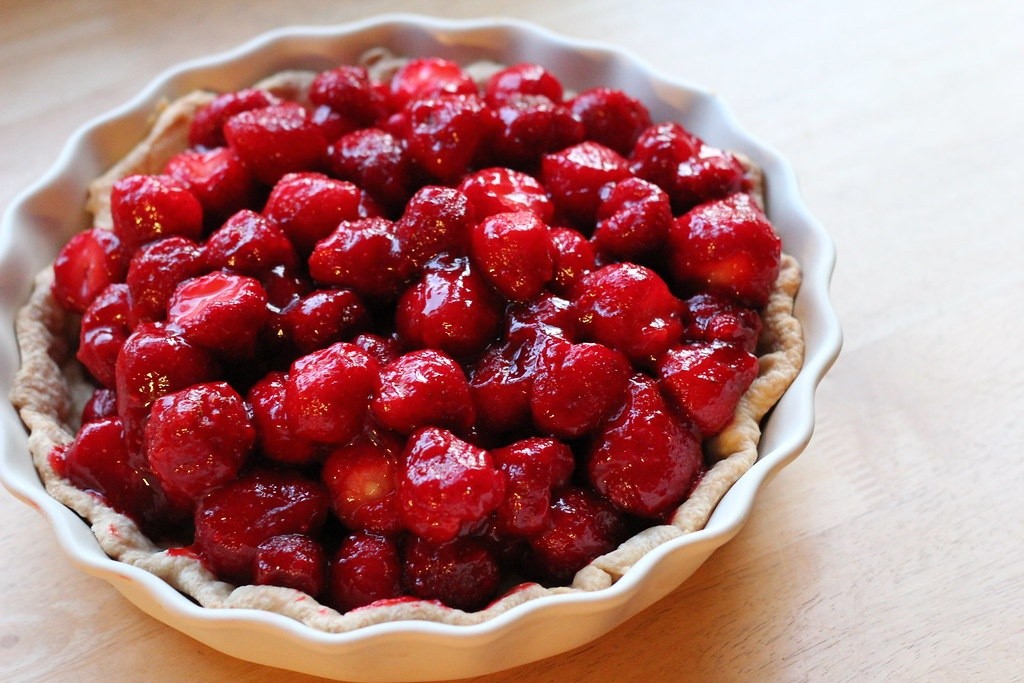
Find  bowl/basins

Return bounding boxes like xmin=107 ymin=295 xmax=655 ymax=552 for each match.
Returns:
xmin=0 ymin=14 xmax=844 ymax=683
